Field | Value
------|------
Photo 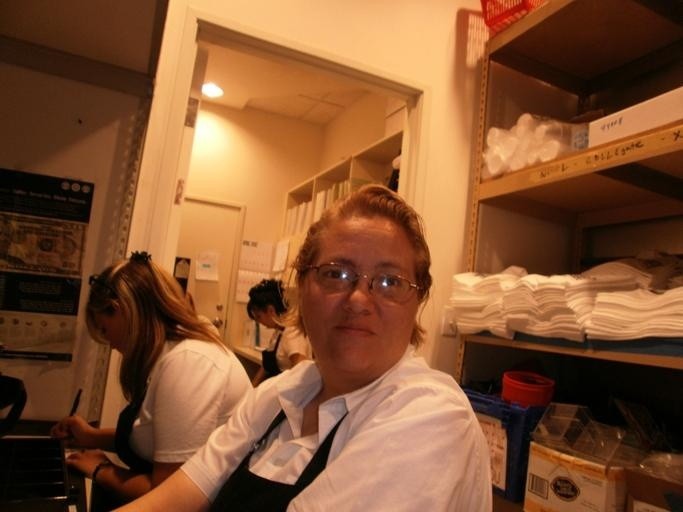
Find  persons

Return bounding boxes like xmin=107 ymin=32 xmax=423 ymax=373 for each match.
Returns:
xmin=99 ymin=181 xmax=493 ymax=512
xmin=246 ymin=275 xmax=313 ymax=389
xmin=49 ymin=257 xmax=253 ymax=500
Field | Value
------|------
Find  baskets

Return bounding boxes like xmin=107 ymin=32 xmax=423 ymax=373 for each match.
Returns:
xmin=480 ymin=0 xmax=545 ymax=32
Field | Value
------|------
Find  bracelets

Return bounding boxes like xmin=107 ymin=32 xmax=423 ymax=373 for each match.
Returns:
xmin=91 ymin=460 xmax=113 ymax=481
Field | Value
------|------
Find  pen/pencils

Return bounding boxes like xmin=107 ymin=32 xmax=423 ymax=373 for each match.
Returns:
xmin=62 ymin=389 xmax=82 ymax=432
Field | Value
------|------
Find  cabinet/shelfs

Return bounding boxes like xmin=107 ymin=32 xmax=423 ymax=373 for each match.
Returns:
xmin=273 ymin=129 xmax=403 ymax=289
xmin=454 ymin=1 xmax=683 ymax=511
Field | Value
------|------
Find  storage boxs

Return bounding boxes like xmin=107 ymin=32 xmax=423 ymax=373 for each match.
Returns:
xmin=459 ymin=382 xmax=682 ymax=511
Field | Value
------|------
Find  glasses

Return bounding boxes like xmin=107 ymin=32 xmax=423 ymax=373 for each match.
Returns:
xmin=303 ymin=260 xmax=420 ymax=302
